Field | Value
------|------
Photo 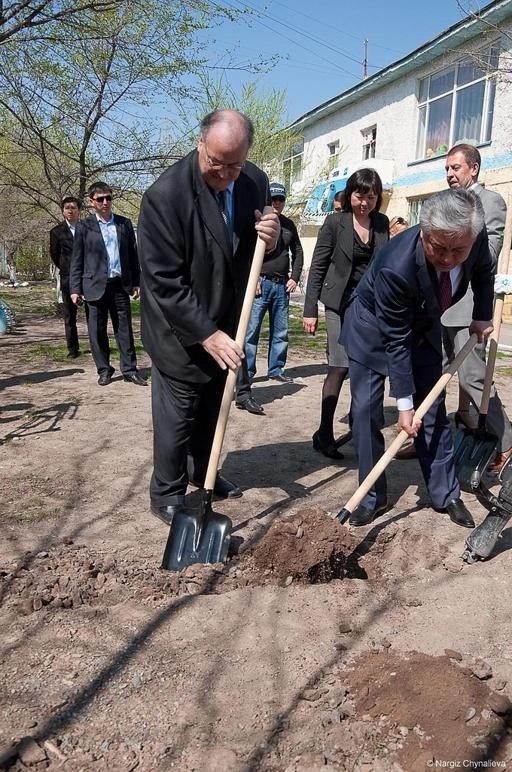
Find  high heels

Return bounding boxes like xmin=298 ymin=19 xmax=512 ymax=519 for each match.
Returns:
xmin=453 ymin=409 xmax=478 ymax=430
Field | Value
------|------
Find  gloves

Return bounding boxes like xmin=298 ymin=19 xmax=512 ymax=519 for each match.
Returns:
xmin=494 ymin=273 xmax=512 ymax=295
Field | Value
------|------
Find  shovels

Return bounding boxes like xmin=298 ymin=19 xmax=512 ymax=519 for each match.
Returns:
xmin=453 ymin=194 xmax=512 ymax=493
xmin=161 ymin=206 xmax=274 ymax=571
xmin=253 ymin=332 xmax=478 ymax=582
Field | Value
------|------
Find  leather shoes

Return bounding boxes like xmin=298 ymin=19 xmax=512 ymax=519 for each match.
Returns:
xmin=270 ymin=375 xmax=295 ymax=383
xmin=98 ymin=371 xmax=112 ymax=385
xmin=444 ymin=497 xmax=477 ymax=529
xmin=123 ymin=373 xmax=148 ymax=386
xmin=313 ymin=429 xmax=345 ymax=462
xmin=192 ymin=472 xmax=244 ymax=499
xmin=394 ymin=443 xmax=417 ymax=460
xmin=150 ymin=503 xmax=177 ymax=526
xmin=235 ymin=397 xmax=265 ymax=413
xmin=66 ymin=351 xmax=79 ymax=359
xmin=348 ymin=493 xmax=389 ymax=528
xmin=487 ymin=446 xmax=512 ymax=473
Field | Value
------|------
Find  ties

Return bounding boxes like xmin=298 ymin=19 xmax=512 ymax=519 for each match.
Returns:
xmin=437 ymin=270 xmax=452 ymax=313
xmin=218 ymin=190 xmax=229 ymax=230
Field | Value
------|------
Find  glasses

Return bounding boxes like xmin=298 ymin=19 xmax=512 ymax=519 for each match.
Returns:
xmin=92 ymin=195 xmax=113 ymax=203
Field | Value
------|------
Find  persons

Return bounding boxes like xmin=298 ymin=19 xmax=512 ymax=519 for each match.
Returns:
xmin=337 ymin=188 xmax=494 ymax=527
xmin=303 ymin=168 xmax=389 ymax=460
xmin=236 ymin=354 xmax=264 ymax=413
xmin=137 ymin=109 xmax=281 ymax=524
xmin=70 ymin=181 xmax=148 ymax=386
xmin=389 ymin=216 xmax=409 ymax=241
xmin=50 ymin=197 xmax=83 ymax=359
xmin=246 ymin=183 xmax=303 ymax=385
xmin=455 ymin=390 xmax=477 ymax=431
xmin=334 ymin=191 xmax=346 ymax=214
xmin=394 ymin=144 xmax=512 ymax=476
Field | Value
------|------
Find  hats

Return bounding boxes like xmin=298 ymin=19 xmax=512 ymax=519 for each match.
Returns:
xmin=268 ymin=182 xmax=288 ymax=198
